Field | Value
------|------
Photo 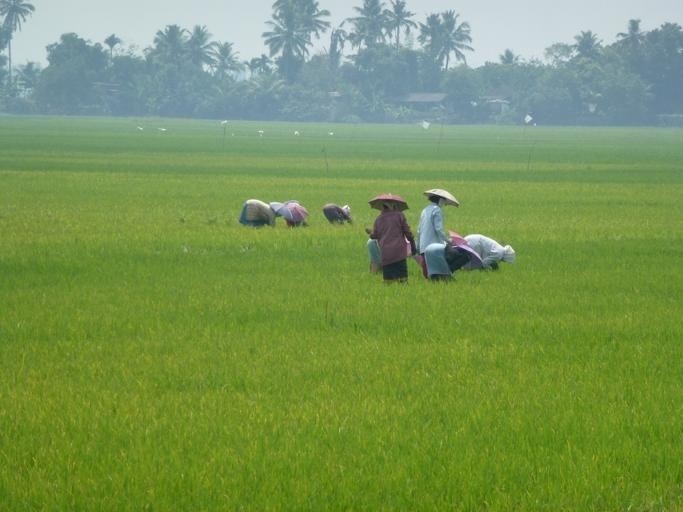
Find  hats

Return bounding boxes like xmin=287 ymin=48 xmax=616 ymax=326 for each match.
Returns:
xmin=448 ymin=231 xmax=468 ymax=246
xmin=288 ymin=204 xmax=308 ymax=221
xmin=270 ymin=202 xmax=291 ymax=219
xmin=455 ymin=245 xmax=483 ymax=269
xmin=423 ymin=189 xmax=460 ymax=207
xmin=369 ymin=193 xmax=408 ymax=211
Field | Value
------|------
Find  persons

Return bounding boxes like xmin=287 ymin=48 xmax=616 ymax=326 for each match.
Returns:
xmin=283 ymin=202 xmax=309 ymax=226
xmin=239 ymin=199 xmax=285 ymax=227
xmin=423 ymin=240 xmax=474 ymax=281
xmin=366 ymin=236 xmax=411 ymax=274
xmin=365 ymin=201 xmax=416 ymax=283
xmin=462 ymin=233 xmax=514 ymax=272
xmin=415 ymin=195 xmax=453 ymax=281
xmin=322 ymin=203 xmax=352 ymax=225
